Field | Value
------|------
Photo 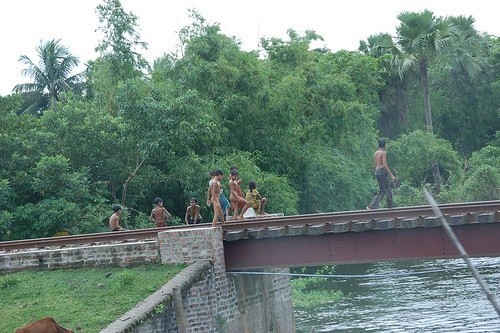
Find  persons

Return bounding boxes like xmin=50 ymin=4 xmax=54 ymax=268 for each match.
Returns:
xmin=206 ymin=166 xmax=249 ymax=226
xmin=245 ymin=181 xmax=267 ymax=214
xmin=110 ymin=206 xmax=126 ymax=231
xmin=149 ymin=197 xmax=170 ymax=227
xmin=365 ymin=140 xmax=396 ymax=208
xmin=185 ymin=197 xmax=202 ymax=225
